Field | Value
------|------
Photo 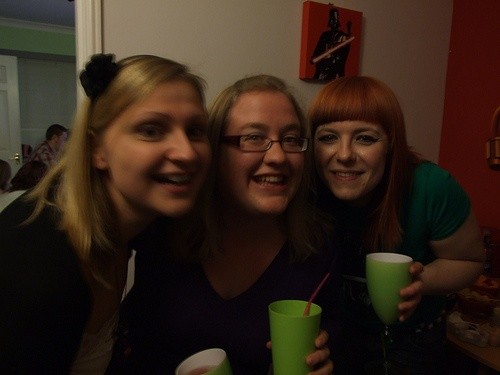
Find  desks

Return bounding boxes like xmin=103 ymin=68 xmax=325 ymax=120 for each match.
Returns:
xmin=448 ymin=317 xmax=500 ymax=375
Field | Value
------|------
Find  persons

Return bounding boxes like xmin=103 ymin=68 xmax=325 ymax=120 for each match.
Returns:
xmin=312 ymin=8 xmax=354 ymax=80
xmin=303 ymin=76 xmax=488 ymax=375
xmin=0 ymin=52 xmax=336 ymax=375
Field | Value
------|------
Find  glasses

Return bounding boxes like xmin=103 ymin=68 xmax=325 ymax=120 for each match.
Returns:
xmin=219 ymin=134 xmax=311 ymax=153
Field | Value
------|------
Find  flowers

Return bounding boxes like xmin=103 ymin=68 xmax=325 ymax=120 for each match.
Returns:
xmin=79 ymin=54 xmax=120 ymax=102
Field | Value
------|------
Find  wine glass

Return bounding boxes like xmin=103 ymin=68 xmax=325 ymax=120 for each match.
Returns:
xmin=365 ymin=251 xmax=413 ymax=375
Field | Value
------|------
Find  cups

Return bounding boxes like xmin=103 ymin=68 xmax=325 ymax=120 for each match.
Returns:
xmin=267 ymin=299 xmax=323 ymax=375
xmin=173 ymin=348 xmax=232 ymax=375
